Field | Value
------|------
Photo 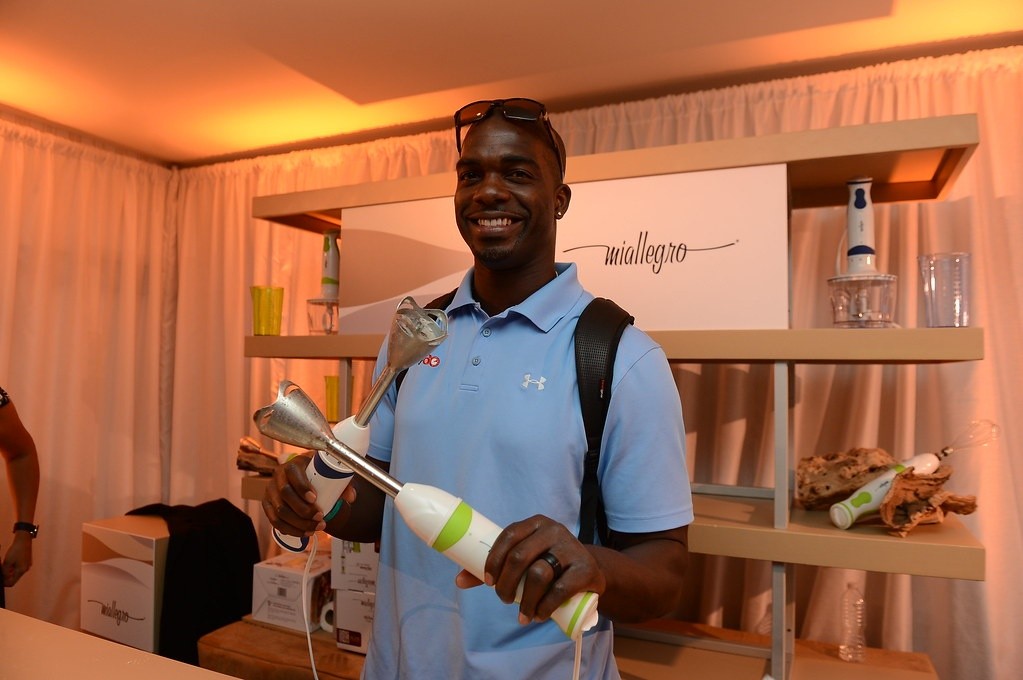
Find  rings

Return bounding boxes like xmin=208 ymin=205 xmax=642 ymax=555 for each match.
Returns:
xmin=538 ymin=552 xmax=562 ymax=576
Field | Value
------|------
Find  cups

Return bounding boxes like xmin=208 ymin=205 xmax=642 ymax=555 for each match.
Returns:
xmin=919 ymin=249 xmax=974 ymax=329
xmin=249 ymin=285 xmax=284 ymax=336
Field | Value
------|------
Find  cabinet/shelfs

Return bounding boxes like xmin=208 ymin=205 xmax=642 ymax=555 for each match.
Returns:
xmin=244 ymin=111 xmax=986 ymax=679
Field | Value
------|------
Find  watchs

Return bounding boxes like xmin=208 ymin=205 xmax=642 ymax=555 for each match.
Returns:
xmin=13 ymin=521 xmax=41 ymax=539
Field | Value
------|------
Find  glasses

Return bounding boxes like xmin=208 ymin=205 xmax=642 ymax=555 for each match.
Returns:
xmin=454 ymin=97 xmax=564 ymax=181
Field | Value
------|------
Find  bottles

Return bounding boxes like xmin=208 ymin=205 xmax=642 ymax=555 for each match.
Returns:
xmin=836 ymin=579 xmax=868 ymax=662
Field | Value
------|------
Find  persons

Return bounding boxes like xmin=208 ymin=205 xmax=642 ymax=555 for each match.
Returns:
xmin=258 ymin=96 xmax=694 ymax=680
xmin=0 ymin=386 xmax=40 ymax=610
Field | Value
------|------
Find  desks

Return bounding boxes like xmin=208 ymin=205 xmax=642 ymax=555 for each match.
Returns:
xmin=0 ymin=607 xmax=247 ymax=680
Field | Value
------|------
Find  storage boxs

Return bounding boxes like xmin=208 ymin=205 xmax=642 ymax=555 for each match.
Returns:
xmin=80 ymin=515 xmax=171 ymax=653
xmin=252 ymin=531 xmax=377 ymax=652
